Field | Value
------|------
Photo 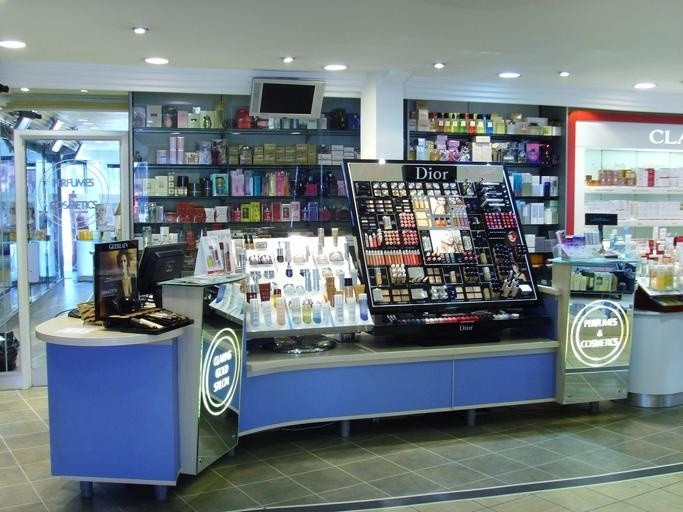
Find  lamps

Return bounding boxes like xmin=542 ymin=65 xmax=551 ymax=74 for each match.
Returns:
xmin=50 ymin=116 xmax=62 ymax=130
xmin=8 ymin=115 xmax=31 ymax=130
xmin=49 ymin=139 xmax=62 ymax=152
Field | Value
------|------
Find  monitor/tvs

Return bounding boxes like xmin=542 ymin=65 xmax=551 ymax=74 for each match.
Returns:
xmin=138 ymin=241 xmax=188 ymax=294
xmin=249 ymin=79 xmax=326 ymax=120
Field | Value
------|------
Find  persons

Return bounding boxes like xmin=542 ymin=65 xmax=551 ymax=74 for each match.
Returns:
xmin=106 ymin=249 xmax=137 ymax=307
xmin=95 ymin=204 xmax=113 ymax=227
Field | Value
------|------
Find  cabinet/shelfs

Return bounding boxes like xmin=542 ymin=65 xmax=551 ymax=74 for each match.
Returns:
xmin=403 ymin=99 xmax=567 ymax=284
xmin=576 ymin=121 xmax=683 ymax=244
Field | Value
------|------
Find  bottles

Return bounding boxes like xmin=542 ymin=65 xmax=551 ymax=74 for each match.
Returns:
xmin=428 ymin=111 xmax=493 ymax=134
